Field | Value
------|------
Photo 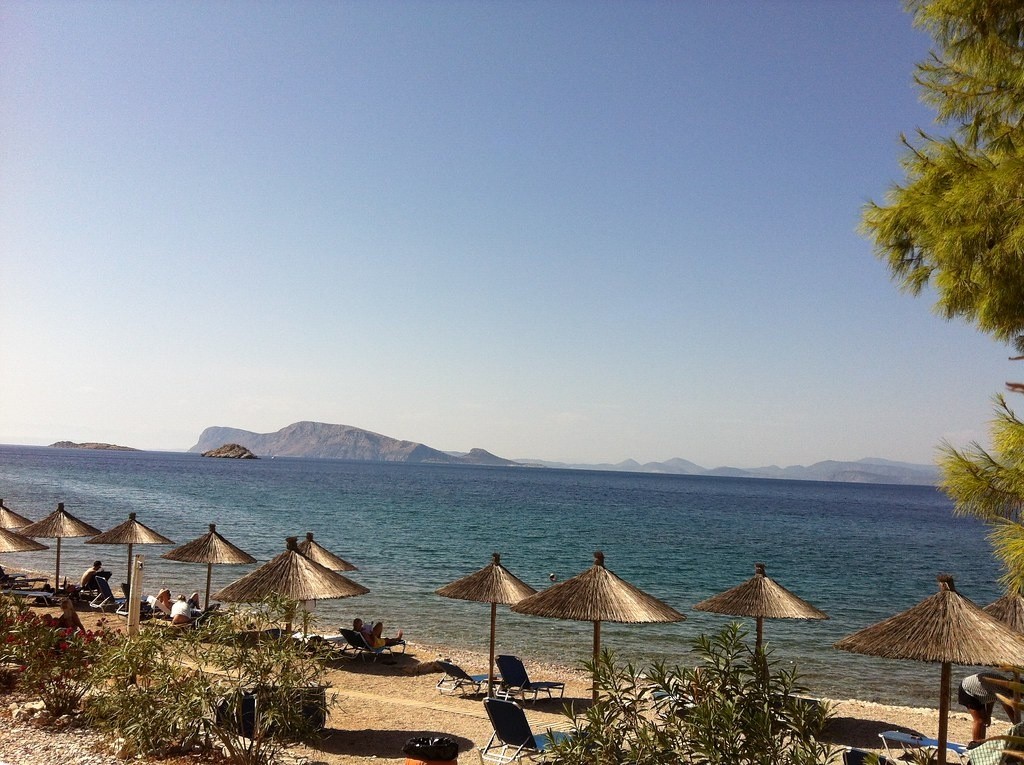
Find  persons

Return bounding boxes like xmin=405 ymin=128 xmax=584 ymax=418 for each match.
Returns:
xmin=957 ymin=673 xmax=1020 ymax=748
xmin=157 ymin=588 xmax=200 ymax=624
xmin=352 ymin=616 xmax=406 ymax=651
xmin=80 ymin=561 xmax=103 ymax=593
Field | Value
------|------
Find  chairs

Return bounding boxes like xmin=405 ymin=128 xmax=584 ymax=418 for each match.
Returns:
xmin=878 ymin=730 xmax=1024 ymax=765
xmin=478 ymin=697 xmax=578 ymax=765
xmin=495 ymin=655 xmax=565 ymax=707
xmin=0 ymin=568 xmax=221 ymax=628
xmin=436 ymin=659 xmax=501 ymax=696
xmin=337 ymin=628 xmax=406 ymax=662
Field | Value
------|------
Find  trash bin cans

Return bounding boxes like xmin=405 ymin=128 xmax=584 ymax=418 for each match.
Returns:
xmin=405 ymin=737 xmax=458 ymax=765
xmin=290 ymin=686 xmax=326 ymax=733
xmin=236 ymin=688 xmax=274 ymax=739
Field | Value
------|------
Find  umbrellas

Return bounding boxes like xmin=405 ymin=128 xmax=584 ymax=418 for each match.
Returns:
xmin=830 ymin=577 xmax=1023 ymax=764
xmin=166 ymin=522 xmax=371 ymax=636
xmin=691 ymin=564 xmax=828 ymax=698
xmin=1 ymin=499 xmax=177 ymax=615
xmin=436 ymin=551 xmax=687 ymax=716
